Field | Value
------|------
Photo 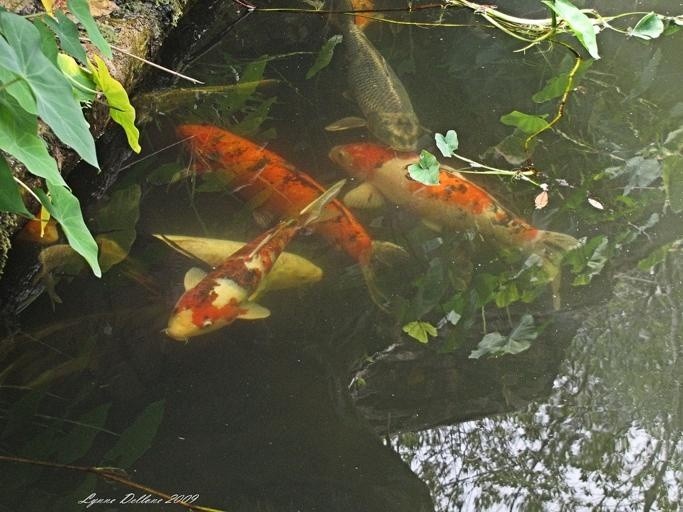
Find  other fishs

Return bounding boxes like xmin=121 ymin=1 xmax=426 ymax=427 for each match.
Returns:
xmin=158 ymin=178 xmax=348 ymax=345
